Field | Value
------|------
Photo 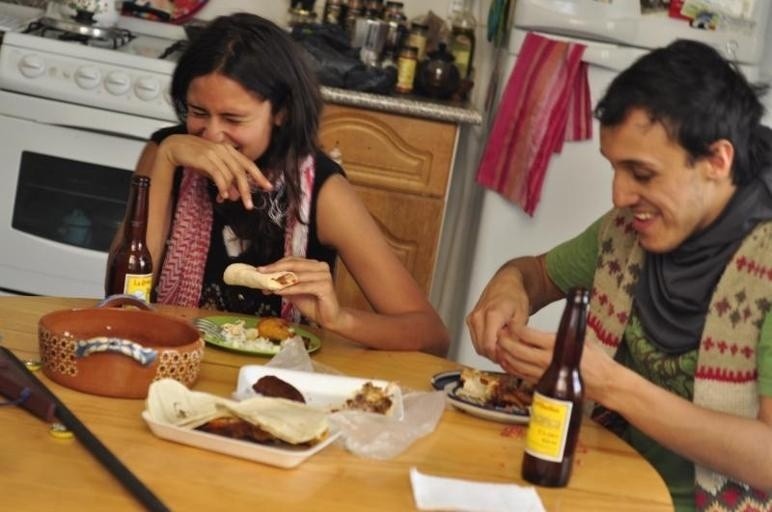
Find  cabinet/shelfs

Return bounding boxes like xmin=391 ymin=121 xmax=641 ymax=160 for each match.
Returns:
xmin=313 ymin=96 xmax=457 ymax=315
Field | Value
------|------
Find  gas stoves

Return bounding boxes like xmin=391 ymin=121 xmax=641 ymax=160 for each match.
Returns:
xmin=0 ymin=14 xmax=194 ymax=126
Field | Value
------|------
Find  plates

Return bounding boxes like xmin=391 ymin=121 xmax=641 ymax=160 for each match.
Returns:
xmin=431 ymin=368 xmax=532 ymax=423
xmin=193 ymin=314 xmax=322 ymax=356
xmin=140 ymin=394 xmax=348 ymax=469
xmin=233 ymin=362 xmax=404 ymax=429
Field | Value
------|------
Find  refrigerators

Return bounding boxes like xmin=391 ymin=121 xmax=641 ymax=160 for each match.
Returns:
xmin=433 ymin=1 xmax=771 ymax=369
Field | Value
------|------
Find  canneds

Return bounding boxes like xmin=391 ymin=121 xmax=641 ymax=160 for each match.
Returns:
xmin=289 ymin=0 xmax=430 ymax=93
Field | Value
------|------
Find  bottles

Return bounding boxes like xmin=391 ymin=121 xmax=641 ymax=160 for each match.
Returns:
xmin=521 ymin=286 xmax=592 ymax=488
xmin=285 ymin=0 xmax=474 ymax=102
xmin=105 ymin=177 xmax=156 ymax=308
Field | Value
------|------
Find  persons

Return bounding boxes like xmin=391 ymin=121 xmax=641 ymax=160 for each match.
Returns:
xmin=461 ymin=36 xmax=771 ymax=512
xmin=103 ymin=11 xmax=453 ymax=362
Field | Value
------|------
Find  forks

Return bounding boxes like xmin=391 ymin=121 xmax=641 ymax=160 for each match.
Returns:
xmin=190 ymin=315 xmax=227 ymax=340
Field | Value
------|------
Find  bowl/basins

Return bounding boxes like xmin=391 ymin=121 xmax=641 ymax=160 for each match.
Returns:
xmin=38 ymin=294 xmax=207 ymax=401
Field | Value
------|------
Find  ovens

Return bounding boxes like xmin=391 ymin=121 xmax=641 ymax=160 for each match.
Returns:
xmin=1 ymin=91 xmax=183 ymax=308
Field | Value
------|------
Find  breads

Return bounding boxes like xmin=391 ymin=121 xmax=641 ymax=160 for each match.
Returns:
xmin=215 ymin=397 xmax=330 ymax=445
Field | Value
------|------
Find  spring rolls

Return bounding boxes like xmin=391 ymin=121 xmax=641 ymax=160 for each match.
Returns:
xmin=223 ymin=263 xmax=298 ymax=291
